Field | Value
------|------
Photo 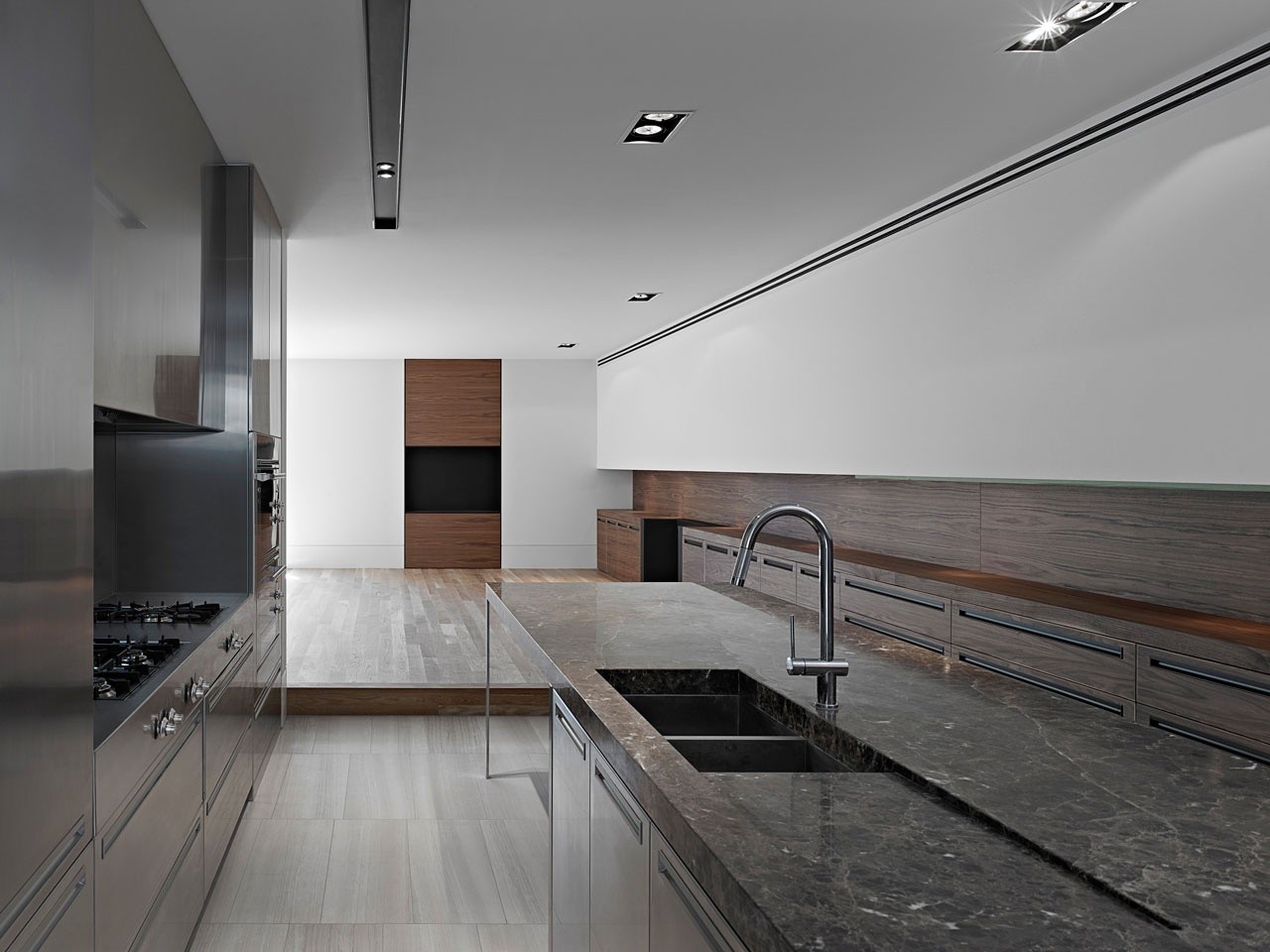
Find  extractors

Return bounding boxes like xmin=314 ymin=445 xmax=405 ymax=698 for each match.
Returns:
xmin=92 ymin=3 xmax=226 ymax=435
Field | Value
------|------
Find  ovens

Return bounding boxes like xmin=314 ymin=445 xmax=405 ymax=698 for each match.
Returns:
xmin=248 ymin=431 xmax=287 ymax=715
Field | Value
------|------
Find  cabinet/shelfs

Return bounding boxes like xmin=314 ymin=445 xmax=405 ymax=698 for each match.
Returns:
xmin=99 ymin=634 xmax=250 ymax=951
xmin=542 ymin=681 xmax=649 ymax=952
xmin=255 ymin=576 xmax=286 ymax=781
xmin=597 ymin=517 xmax=617 ymax=576
xmin=642 ymin=817 xmax=751 ymax=952
xmin=222 ymin=161 xmax=288 ymax=439
xmin=1 ymin=5 xmax=97 ymax=952
xmin=616 ymin=521 xmax=642 ymax=584
xmin=676 ymin=522 xmax=1270 ymax=771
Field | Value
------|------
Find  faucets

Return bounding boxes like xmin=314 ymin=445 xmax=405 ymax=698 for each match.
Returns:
xmin=730 ymin=504 xmax=850 ymax=710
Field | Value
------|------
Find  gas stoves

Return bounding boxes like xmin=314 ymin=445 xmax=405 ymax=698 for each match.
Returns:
xmin=93 ymin=592 xmax=254 ymax=837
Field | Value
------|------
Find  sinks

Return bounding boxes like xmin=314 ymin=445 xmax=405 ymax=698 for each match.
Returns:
xmin=597 ymin=668 xmax=808 ymax=738
xmin=664 ymin=734 xmax=905 ymax=773
